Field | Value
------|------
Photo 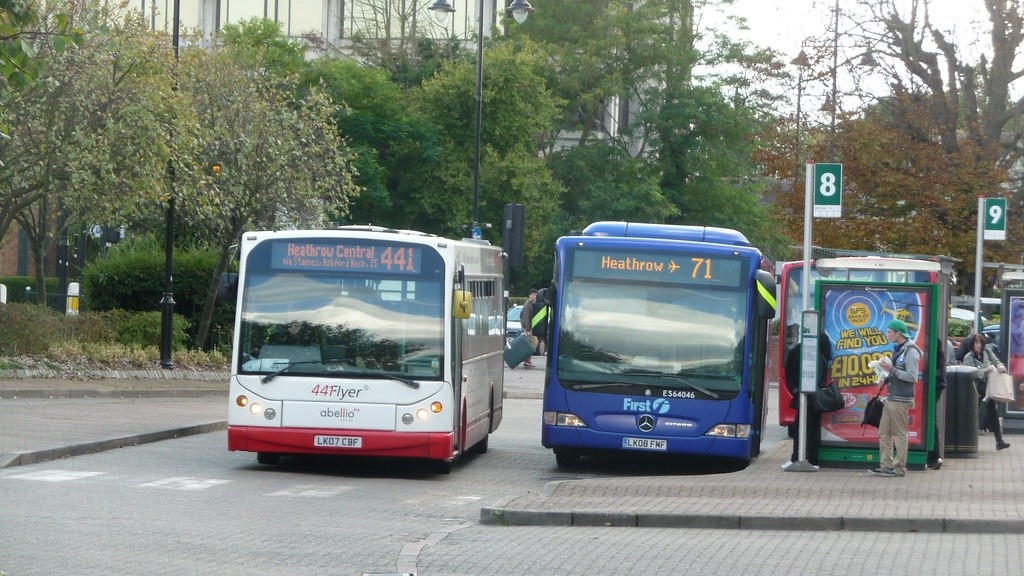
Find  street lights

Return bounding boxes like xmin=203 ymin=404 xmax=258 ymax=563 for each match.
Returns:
xmin=791 ymin=41 xmax=881 ymax=162
xmin=428 ymin=0 xmax=538 ymax=238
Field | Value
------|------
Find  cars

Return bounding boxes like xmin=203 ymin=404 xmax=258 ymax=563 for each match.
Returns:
xmin=949 ymin=308 xmax=1003 ymax=352
xmin=504 ymin=305 xmax=546 ymax=357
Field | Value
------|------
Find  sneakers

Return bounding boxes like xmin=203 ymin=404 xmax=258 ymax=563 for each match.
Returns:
xmin=780 ymin=461 xmax=793 ymax=469
xmin=814 ymin=466 xmax=819 ymax=470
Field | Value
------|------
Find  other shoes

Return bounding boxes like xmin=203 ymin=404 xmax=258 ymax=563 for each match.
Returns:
xmin=995 ymin=440 xmax=1010 ymax=450
xmin=524 ymin=362 xmax=536 ymax=367
xmin=881 ymin=468 xmax=897 ymax=476
xmin=868 ymin=466 xmax=882 ymax=475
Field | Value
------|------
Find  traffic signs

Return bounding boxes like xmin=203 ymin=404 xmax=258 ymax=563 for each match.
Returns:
xmin=90 ymin=225 xmax=124 ymax=238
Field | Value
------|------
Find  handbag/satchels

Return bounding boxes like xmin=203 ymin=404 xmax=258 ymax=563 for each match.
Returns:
xmin=982 ymin=366 xmax=1015 ymax=403
xmin=812 ymin=382 xmax=845 ymax=412
xmin=862 ymin=397 xmax=885 ymax=427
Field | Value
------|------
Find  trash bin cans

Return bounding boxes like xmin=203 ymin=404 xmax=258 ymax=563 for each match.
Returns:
xmin=983 ymin=325 xmax=1001 ymax=348
xmin=945 ymin=365 xmax=981 ymax=458
xmin=66 ymin=282 xmax=80 ymax=315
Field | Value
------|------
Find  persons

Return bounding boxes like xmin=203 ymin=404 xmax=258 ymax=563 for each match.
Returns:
xmin=946 ymin=339 xmax=962 ymax=366
xmin=964 ymin=333 xmax=1010 ymax=450
xmin=926 ymin=337 xmax=946 ymax=470
xmin=781 ymin=332 xmax=831 ymax=469
xmin=520 ymin=288 xmax=537 ymax=366
xmin=955 ymin=321 xmax=1002 ymax=433
xmin=867 ymin=320 xmax=920 ymax=477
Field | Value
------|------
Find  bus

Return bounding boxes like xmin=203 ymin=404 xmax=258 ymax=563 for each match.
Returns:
xmin=217 ymin=226 xmax=507 ymax=474
xmin=778 ymin=255 xmax=953 ymax=440
xmin=528 ymin=220 xmax=789 ymax=473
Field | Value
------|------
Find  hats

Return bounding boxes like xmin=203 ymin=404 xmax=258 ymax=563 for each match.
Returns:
xmin=887 ymin=319 xmax=912 ymax=339
